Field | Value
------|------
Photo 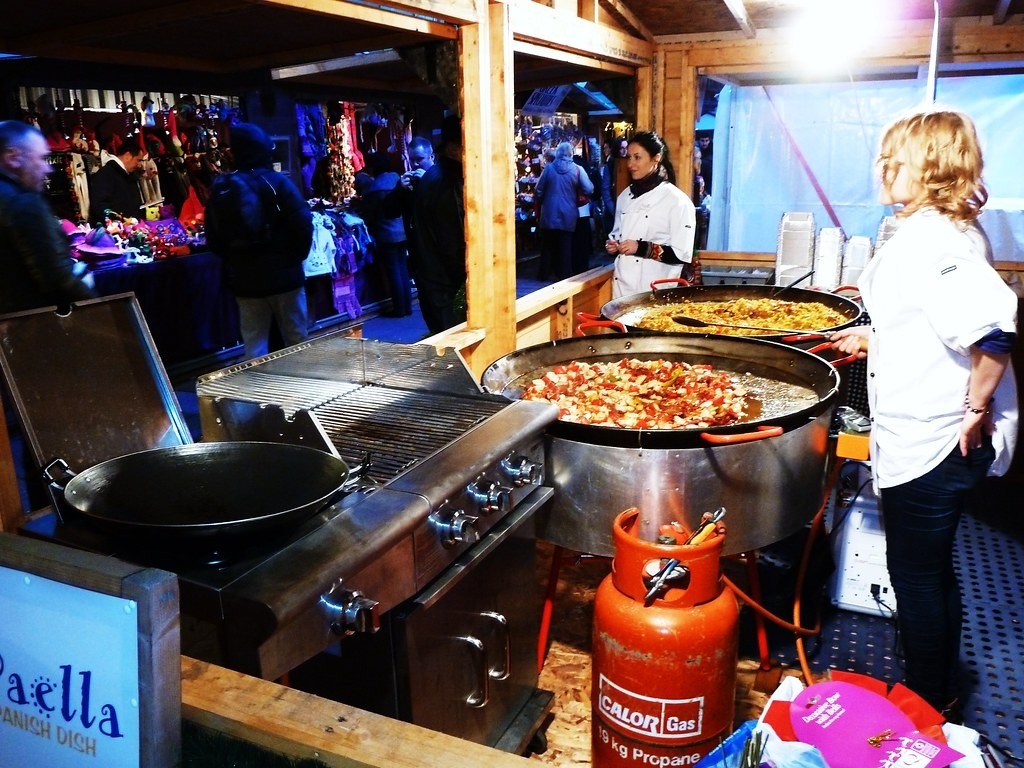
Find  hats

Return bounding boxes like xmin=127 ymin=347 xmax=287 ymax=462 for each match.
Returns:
xmin=230 ymin=124 xmax=279 ymax=160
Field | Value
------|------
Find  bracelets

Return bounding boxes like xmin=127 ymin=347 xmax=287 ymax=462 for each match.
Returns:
xmin=964 ymin=389 xmax=997 ymax=415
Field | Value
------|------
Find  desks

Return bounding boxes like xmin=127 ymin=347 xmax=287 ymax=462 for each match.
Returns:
xmin=85 ymin=240 xmax=390 ymax=370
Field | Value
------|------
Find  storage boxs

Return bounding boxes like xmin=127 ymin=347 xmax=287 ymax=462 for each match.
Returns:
xmin=776 ymin=212 xmax=900 ymax=298
xmin=701 ymin=265 xmax=776 ymax=285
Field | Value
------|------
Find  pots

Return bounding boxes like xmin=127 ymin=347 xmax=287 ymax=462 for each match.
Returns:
xmin=578 ymin=280 xmax=863 ymax=341
xmin=480 ymin=321 xmax=841 ymax=449
xmin=43 ymin=441 xmax=373 ymax=560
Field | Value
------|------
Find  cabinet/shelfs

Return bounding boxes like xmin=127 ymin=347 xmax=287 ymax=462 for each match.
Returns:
xmin=514 ymin=110 xmax=589 ymax=261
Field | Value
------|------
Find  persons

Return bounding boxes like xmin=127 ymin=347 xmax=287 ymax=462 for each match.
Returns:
xmin=827 ymin=112 xmax=1020 ymax=723
xmin=0 ymin=120 xmax=713 ymax=517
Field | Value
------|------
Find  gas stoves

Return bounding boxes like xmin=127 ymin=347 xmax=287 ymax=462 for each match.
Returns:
xmin=0 ymin=292 xmax=559 ymax=682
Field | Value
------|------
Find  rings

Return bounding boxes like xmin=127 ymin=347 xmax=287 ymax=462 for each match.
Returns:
xmin=605 ymin=244 xmax=609 ymax=249
